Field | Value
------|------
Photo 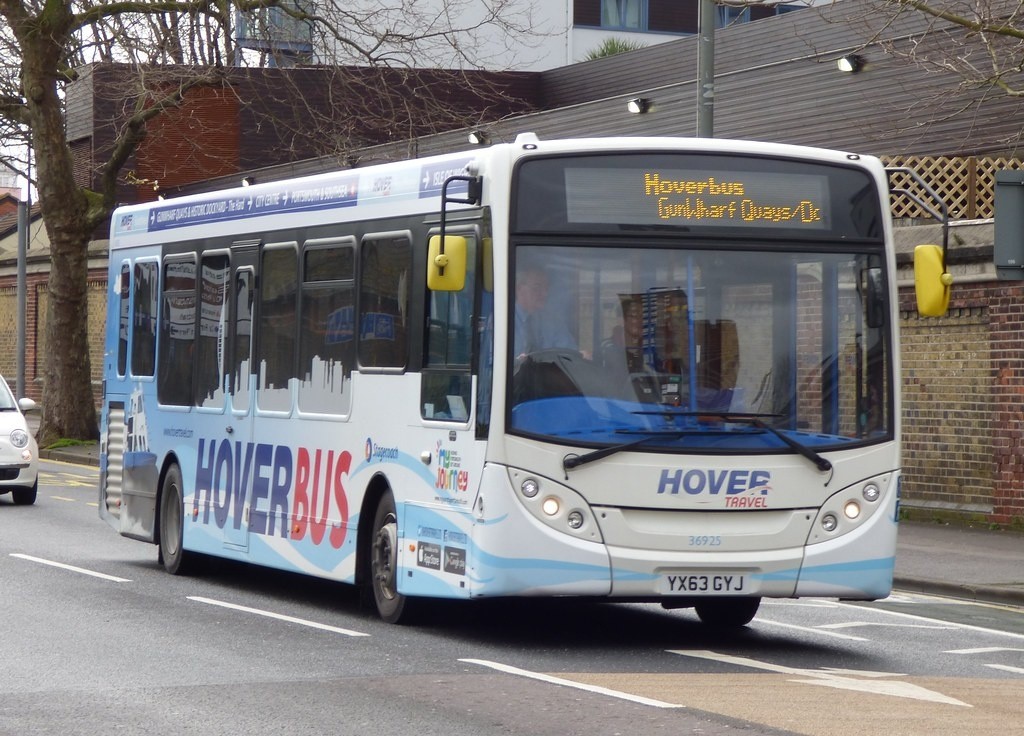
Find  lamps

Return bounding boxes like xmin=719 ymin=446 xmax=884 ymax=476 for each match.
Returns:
xmin=626 ymin=97 xmax=645 ymax=113
xmin=836 ymin=54 xmax=858 ymax=72
xmin=158 ymin=193 xmax=167 ymax=200
xmin=467 ymin=131 xmax=483 ymax=144
xmin=241 ymin=176 xmax=253 ymax=187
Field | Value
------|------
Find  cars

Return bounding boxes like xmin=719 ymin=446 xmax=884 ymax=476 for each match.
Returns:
xmin=0 ymin=372 xmax=42 ymax=505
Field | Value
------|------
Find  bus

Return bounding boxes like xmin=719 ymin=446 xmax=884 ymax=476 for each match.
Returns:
xmin=95 ymin=127 xmax=957 ymax=630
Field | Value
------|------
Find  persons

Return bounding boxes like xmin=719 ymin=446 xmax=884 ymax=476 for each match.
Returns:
xmin=600 ymin=296 xmax=680 ymax=385
xmin=476 ymin=261 xmax=595 ymax=425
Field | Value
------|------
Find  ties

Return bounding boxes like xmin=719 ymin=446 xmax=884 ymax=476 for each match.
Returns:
xmin=524 ymin=316 xmax=537 ymax=355
